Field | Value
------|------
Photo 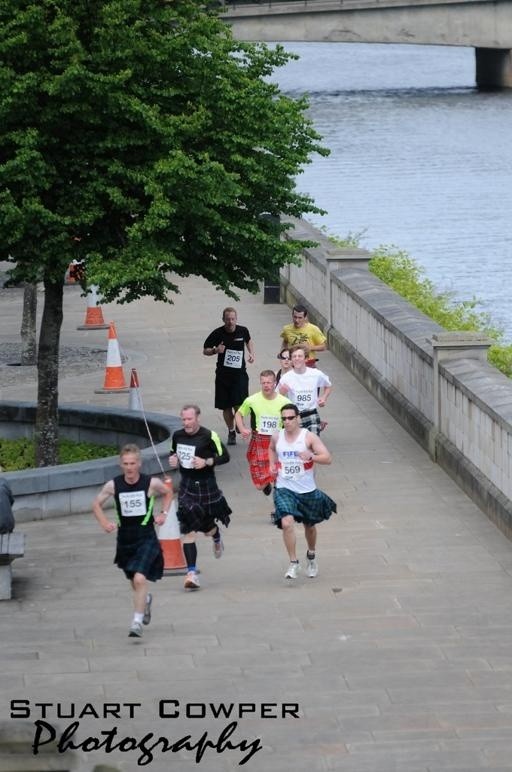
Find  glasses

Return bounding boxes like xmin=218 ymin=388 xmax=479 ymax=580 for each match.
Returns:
xmin=281 ymin=416 xmax=296 ymax=421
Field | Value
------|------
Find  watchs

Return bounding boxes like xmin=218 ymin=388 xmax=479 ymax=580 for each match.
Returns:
xmin=211 ymin=345 xmax=216 ymax=354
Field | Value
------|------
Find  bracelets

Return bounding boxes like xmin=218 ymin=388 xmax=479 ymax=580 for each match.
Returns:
xmin=160 ymin=509 xmax=169 ymax=518
xmin=307 ymin=453 xmax=314 ymax=461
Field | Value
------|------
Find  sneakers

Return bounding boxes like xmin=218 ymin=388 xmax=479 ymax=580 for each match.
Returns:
xmin=127 ymin=621 xmax=145 ymax=638
xmin=284 ymin=562 xmax=303 ymax=580
xmin=183 ymin=573 xmax=201 ymax=590
xmin=141 ymin=592 xmax=153 ymax=626
xmin=305 ymin=555 xmax=319 ymax=579
xmin=226 ymin=429 xmax=237 ymax=446
xmin=212 ymin=539 xmax=225 ymax=559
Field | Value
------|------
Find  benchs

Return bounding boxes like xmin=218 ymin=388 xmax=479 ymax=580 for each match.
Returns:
xmin=0 ymin=531 xmax=25 ymax=601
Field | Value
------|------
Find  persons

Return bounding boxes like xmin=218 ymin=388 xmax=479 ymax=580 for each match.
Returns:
xmin=279 ymin=305 xmax=328 ymax=365
xmin=203 ymin=307 xmax=255 ymax=444
xmin=281 ymin=369 xmax=281 ymax=370
xmin=235 ymin=370 xmax=293 ymax=496
xmin=92 ymin=442 xmax=174 ymax=637
xmin=168 ymin=404 xmax=232 ymax=589
xmin=268 ymin=404 xmax=337 ymax=578
xmin=0 ymin=477 xmax=14 ymax=533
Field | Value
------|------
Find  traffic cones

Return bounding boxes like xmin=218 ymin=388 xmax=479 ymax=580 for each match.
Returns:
xmin=62 ymin=237 xmax=83 ymax=287
xmin=91 ymin=322 xmax=131 ymax=396
xmin=158 ymin=472 xmax=201 ymax=578
xmin=77 ymin=283 xmax=113 ymax=333
xmin=127 ymin=366 xmax=145 ymax=411
